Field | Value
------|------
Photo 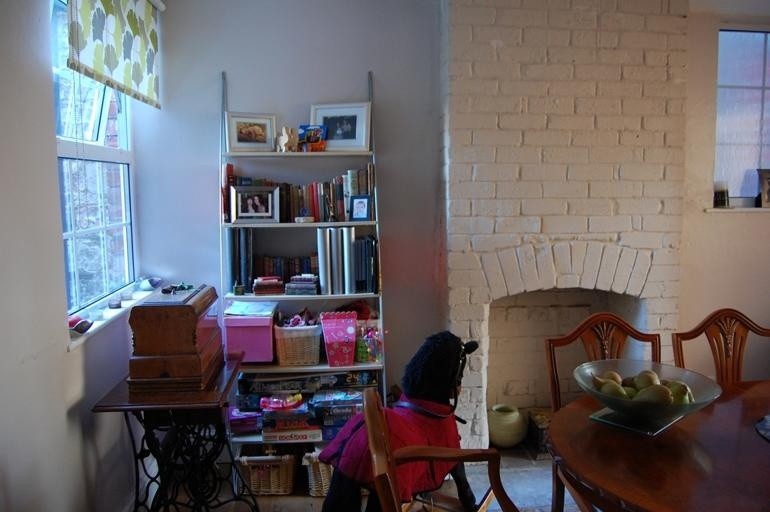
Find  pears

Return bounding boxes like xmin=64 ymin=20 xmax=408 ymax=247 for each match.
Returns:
xmin=590 ymin=370 xmax=695 ymax=405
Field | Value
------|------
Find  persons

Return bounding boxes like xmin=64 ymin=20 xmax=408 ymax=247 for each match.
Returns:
xmin=332 ymin=118 xmax=352 ymax=139
xmin=240 ymin=125 xmax=264 ymax=141
xmin=243 ymin=194 xmax=256 ymax=213
xmin=253 ymin=194 xmax=267 ymax=212
xmin=354 ymin=200 xmax=366 ymax=218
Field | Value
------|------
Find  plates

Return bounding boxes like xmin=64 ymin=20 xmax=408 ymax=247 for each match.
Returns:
xmin=573 ymin=358 xmax=723 ymax=415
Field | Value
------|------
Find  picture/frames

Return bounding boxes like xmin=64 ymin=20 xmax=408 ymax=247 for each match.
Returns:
xmin=349 ymin=194 xmax=372 ymax=221
xmin=224 ymin=111 xmax=277 ymax=152
xmin=310 ymin=101 xmax=372 ymax=152
xmin=230 ymin=185 xmax=280 ymax=224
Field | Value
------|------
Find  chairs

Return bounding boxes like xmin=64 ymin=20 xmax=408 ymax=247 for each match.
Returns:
xmin=671 ymin=308 xmax=770 ymax=383
xmin=545 ymin=311 xmax=661 ymax=512
xmin=362 ymin=388 xmax=519 ymax=512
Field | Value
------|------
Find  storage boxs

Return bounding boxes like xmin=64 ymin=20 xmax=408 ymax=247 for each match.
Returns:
xmin=223 ymin=299 xmax=280 ymax=363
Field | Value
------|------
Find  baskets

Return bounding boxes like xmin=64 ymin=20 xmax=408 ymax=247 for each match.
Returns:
xmin=305 ymin=454 xmax=370 ymax=496
xmin=273 ymin=325 xmax=321 ymax=367
xmin=232 ymin=444 xmax=295 ymax=495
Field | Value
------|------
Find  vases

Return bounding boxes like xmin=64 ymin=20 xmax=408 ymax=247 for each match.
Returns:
xmin=487 ymin=402 xmax=526 ymax=447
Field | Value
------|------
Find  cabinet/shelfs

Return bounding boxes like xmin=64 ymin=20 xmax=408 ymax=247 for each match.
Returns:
xmin=217 ymin=70 xmax=388 ymax=445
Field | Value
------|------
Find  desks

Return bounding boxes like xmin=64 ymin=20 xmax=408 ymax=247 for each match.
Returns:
xmin=91 ymin=350 xmax=260 ymax=512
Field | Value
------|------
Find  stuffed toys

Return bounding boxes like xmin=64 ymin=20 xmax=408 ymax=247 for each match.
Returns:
xmin=317 ymin=330 xmax=479 ymax=512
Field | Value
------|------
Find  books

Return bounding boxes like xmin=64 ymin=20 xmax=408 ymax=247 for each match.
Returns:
xmin=317 ymin=227 xmax=332 ymax=295
xmin=342 ymin=226 xmax=356 ymax=295
xmin=330 ymin=229 xmax=344 ymax=294
xmin=225 ymin=163 xmax=376 ymax=224
xmin=224 ymin=227 xmax=240 ymax=293
xmin=252 ymin=249 xmax=319 ymax=296
xmin=237 ymin=227 xmax=254 ymax=292
xmin=356 ymin=235 xmax=380 ymax=295
